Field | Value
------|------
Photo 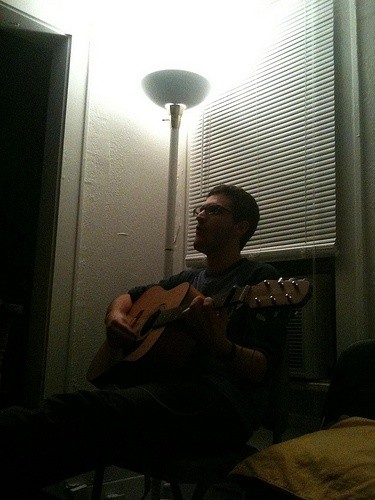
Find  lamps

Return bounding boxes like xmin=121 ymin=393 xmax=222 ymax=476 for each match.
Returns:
xmin=142 ymin=69 xmax=210 ymax=278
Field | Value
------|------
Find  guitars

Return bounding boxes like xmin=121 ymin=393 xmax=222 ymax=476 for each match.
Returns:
xmin=85 ymin=277 xmax=313 ymax=391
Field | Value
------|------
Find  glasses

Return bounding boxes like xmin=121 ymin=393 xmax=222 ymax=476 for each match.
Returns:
xmin=193 ymin=205 xmax=233 ymax=216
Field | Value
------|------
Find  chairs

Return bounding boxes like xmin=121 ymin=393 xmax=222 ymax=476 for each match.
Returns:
xmin=319 ymin=338 xmax=375 ymax=430
xmin=91 ymin=327 xmax=286 ymax=500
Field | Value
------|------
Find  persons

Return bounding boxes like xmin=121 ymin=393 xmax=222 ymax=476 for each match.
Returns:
xmin=0 ymin=184 xmax=289 ymax=500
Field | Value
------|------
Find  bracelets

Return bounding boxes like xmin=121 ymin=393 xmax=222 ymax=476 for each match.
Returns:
xmin=217 ymin=340 xmax=236 ymax=361
